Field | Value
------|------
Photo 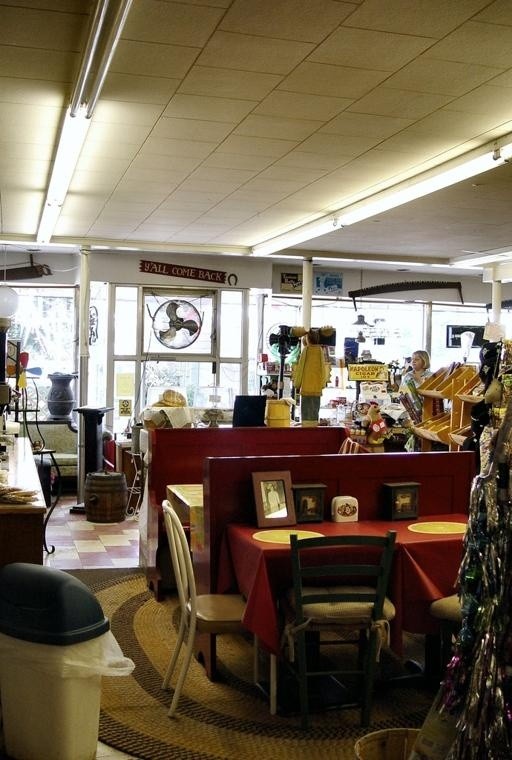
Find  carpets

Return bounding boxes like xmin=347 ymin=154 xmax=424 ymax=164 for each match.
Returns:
xmin=78 ymin=573 xmax=429 ymax=760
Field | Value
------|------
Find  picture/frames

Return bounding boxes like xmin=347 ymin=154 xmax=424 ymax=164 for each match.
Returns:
xmin=251 ymin=469 xmax=297 ymax=528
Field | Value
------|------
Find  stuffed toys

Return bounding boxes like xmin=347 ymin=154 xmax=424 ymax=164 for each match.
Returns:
xmin=361 ymin=401 xmax=387 ymax=445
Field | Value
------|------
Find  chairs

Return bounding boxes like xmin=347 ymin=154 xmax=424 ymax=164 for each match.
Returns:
xmin=286 ymin=528 xmax=398 ymax=725
xmin=161 ymin=500 xmax=257 ymax=717
xmin=421 ymin=593 xmax=475 ymax=704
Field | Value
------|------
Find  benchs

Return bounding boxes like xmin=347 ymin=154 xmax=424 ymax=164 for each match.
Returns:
xmin=19 ymin=420 xmax=78 ymax=478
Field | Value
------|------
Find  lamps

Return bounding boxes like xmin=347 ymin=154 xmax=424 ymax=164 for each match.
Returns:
xmin=36 ymin=13 xmax=131 ymax=247
xmin=249 ymin=133 xmax=510 ymax=259
xmin=1 ymin=235 xmax=19 ymax=318
xmin=352 ymin=265 xmax=374 ymax=343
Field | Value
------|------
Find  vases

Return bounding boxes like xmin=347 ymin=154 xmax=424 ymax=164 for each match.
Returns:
xmin=46 ymin=372 xmax=74 ymax=418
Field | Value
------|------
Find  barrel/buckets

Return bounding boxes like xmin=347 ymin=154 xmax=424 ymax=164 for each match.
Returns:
xmin=84 ymin=471 xmax=128 ymax=523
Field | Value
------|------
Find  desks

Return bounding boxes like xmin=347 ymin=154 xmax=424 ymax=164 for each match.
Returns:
xmin=226 ymin=512 xmax=470 ymax=637
xmin=166 ymin=482 xmax=204 ymax=554
xmin=0 ymin=434 xmax=48 ymax=567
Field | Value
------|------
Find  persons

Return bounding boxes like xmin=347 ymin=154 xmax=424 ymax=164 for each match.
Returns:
xmin=397 ymin=350 xmax=436 ymax=411
xmin=268 ymin=486 xmax=280 ymax=513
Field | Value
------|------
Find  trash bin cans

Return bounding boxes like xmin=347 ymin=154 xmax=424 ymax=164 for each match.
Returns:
xmin=0 ymin=562 xmax=110 ymax=760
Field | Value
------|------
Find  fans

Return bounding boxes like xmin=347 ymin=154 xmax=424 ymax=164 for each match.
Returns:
xmin=152 ymin=299 xmax=202 ymax=349
xmin=266 ymin=322 xmax=300 ymax=402
